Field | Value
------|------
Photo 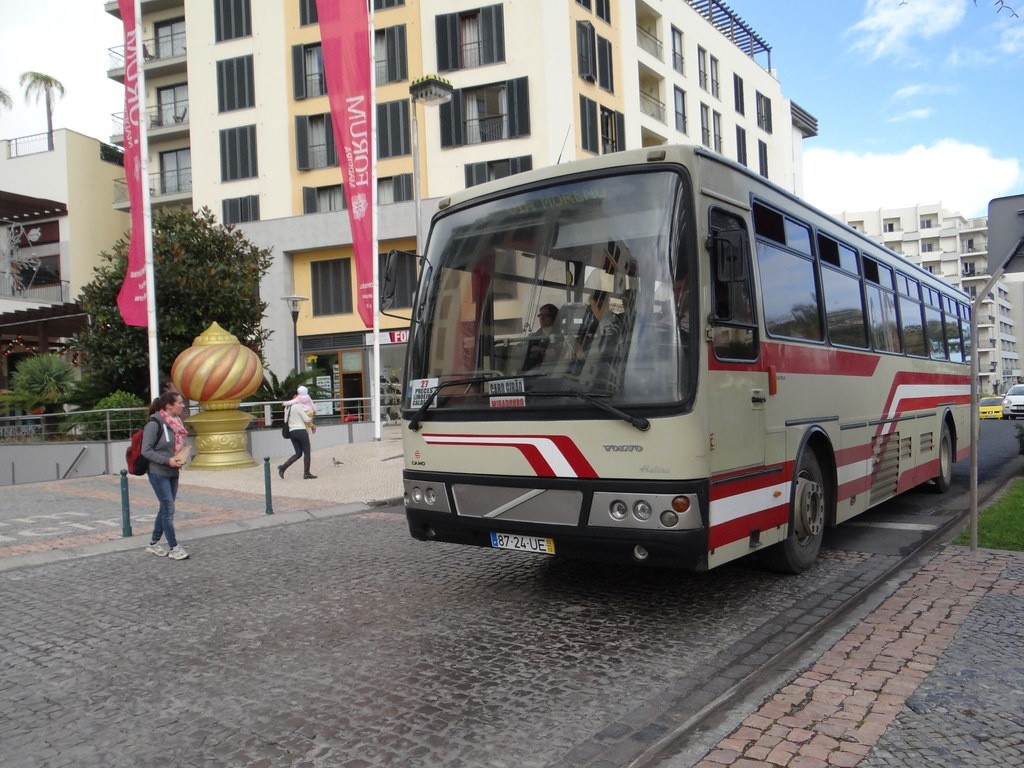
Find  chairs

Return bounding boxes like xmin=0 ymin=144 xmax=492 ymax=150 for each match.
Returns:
xmin=556 ymin=301 xmax=595 ymax=341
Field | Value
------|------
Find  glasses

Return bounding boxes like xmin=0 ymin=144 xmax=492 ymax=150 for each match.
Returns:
xmin=537 ymin=313 xmax=555 ymax=318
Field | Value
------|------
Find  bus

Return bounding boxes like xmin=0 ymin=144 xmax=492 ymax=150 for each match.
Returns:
xmin=383 ymin=142 xmax=977 ymax=575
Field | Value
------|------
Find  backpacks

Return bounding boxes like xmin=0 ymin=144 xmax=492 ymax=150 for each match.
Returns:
xmin=126 ymin=418 xmax=163 ymax=476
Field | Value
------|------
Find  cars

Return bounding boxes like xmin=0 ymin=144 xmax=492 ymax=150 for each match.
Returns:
xmin=979 ymin=397 xmax=1005 ymax=420
xmin=1001 ymin=384 xmax=1024 ymax=420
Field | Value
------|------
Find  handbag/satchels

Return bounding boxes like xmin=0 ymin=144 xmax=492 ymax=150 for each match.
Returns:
xmin=283 ymin=422 xmax=290 ymax=439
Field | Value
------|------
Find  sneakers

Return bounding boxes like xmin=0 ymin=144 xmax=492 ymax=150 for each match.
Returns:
xmin=304 ymin=472 xmax=318 ymax=478
xmin=168 ymin=547 xmax=190 ymax=561
xmin=278 ymin=465 xmax=285 ymax=479
xmin=147 ymin=544 xmax=168 ymax=557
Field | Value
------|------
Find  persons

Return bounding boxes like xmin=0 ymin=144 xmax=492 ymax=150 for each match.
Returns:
xmin=522 ymin=304 xmax=581 ymax=361
xmin=140 ymin=391 xmax=190 ymax=561
xmin=581 ymin=272 xmax=724 ymax=349
xmin=277 ymin=391 xmax=318 ymax=480
xmin=281 ymin=386 xmax=316 ymax=433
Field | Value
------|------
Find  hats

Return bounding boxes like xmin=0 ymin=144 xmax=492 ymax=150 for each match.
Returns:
xmin=297 ymin=386 xmax=308 ymax=395
